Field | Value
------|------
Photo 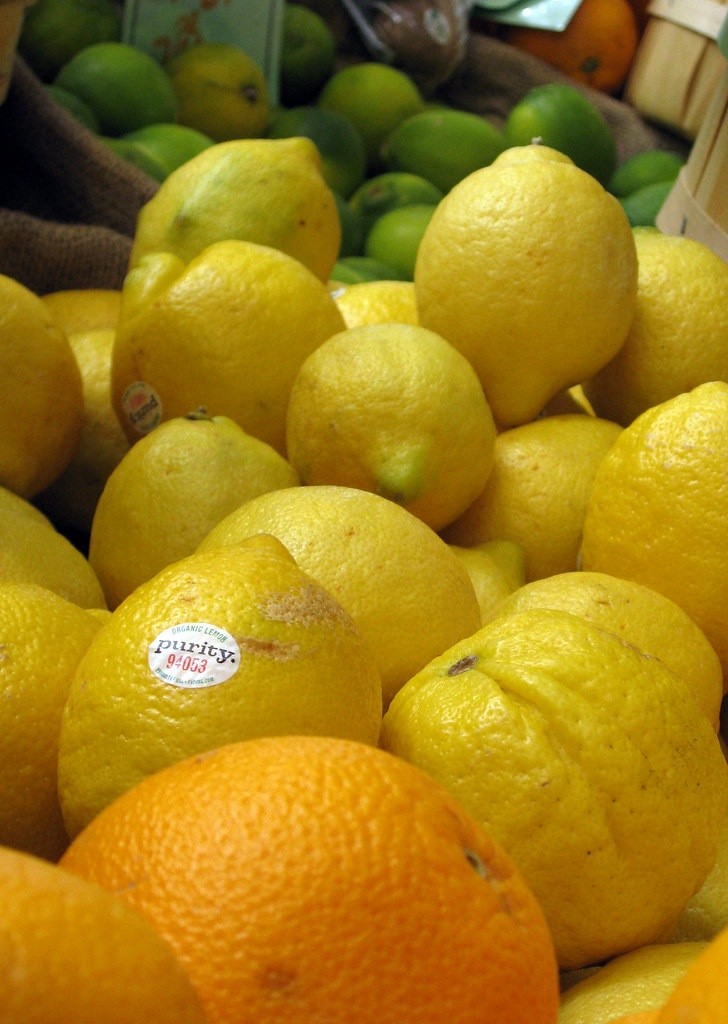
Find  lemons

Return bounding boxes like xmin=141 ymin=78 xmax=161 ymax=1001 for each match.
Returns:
xmin=15 ymin=0 xmax=692 ymax=286
xmin=0 ymin=136 xmax=728 ymax=1024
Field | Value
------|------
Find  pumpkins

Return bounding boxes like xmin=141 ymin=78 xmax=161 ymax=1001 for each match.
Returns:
xmin=506 ymin=0 xmax=639 ymax=90
